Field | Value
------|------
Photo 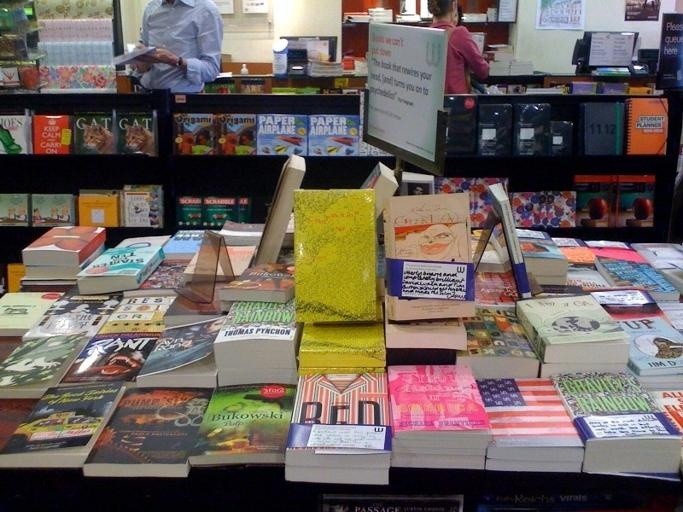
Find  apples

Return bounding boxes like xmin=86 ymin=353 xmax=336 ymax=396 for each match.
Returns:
xmin=587 ymin=198 xmax=608 ymax=219
xmin=632 ymin=197 xmax=651 ymax=220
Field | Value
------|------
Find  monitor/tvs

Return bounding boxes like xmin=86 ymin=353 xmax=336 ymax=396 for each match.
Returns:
xmin=280 ymin=36 xmax=338 ymax=63
xmin=572 ymin=31 xmax=639 ymax=77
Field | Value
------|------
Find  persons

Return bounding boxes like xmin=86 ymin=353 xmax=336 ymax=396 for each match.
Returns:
xmin=127 ymin=0 xmax=225 ymax=95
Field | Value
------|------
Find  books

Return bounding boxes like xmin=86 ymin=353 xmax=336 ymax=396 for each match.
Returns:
xmin=0 ymin=78 xmax=682 ymax=511
xmin=596 ymin=67 xmax=629 ymax=72
xmin=340 ymin=1 xmax=534 ymax=94
xmin=113 ymin=45 xmax=157 ymax=67
xmin=272 ymin=36 xmax=341 ymax=79
xmin=590 ymin=70 xmax=631 ymax=78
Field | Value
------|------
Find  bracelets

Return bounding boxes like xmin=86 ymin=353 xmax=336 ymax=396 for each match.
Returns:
xmin=174 ymin=56 xmax=183 ymax=68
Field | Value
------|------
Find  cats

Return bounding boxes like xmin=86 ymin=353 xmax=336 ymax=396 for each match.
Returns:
xmin=123 ymin=122 xmax=154 ymax=155
xmin=81 ymin=123 xmax=114 ymax=155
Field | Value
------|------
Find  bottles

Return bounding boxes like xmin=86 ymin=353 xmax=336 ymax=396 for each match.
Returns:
xmin=241 ymin=64 xmax=250 ymax=74
xmin=272 ymin=40 xmax=289 ymax=78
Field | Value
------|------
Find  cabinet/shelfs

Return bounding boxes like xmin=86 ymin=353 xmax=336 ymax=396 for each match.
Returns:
xmin=0 ymin=0 xmax=682 ymax=512
